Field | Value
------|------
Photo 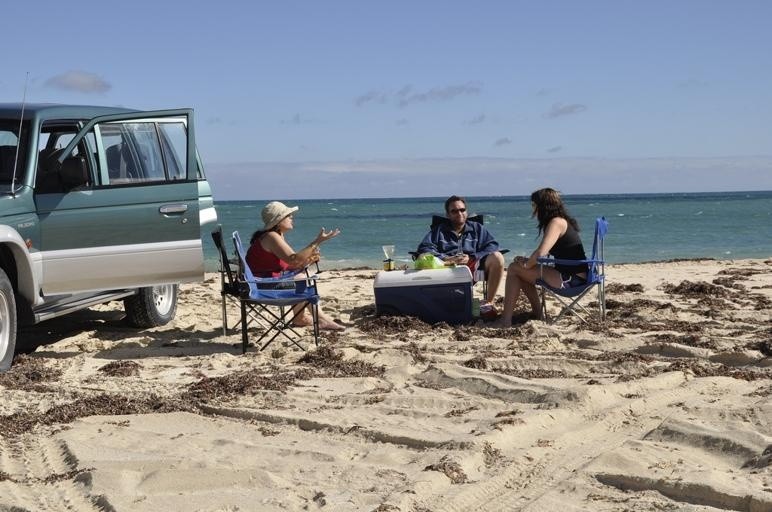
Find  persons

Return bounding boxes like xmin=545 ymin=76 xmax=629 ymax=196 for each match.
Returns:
xmin=487 ymin=187 xmax=589 ymax=327
xmin=243 ymin=201 xmax=347 ymax=333
xmin=415 ymin=195 xmax=505 ymax=318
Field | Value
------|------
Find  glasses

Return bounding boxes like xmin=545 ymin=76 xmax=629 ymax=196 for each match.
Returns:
xmin=450 ymin=208 xmax=466 ymax=215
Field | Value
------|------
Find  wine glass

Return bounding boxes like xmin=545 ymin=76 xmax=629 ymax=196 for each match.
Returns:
xmin=382 ymin=244 xmax=395 ymax=271
xmin=311 ymin=246 xmax=323 ymax=275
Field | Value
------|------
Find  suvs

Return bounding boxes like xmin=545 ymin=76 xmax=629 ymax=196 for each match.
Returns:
xmin=0 ymin=71 xmax=218 ymax=377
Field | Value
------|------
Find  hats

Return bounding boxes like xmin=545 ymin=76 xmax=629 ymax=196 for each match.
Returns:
xmin=262 ymin=200 xmax=299 ymax=231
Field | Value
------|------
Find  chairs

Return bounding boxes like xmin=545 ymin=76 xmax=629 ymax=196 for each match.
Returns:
xmin=232 ymin=230 xmax=320 ymax=354
xmin=408 ymin=215 xmax=510 ymax=300
xmin=210 ymin=224 xmax=285 ymax=337
xmin=536 ymin=216 xmax=606 ymax=327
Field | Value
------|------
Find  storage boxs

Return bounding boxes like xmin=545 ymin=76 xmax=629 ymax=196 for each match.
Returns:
xmin=372 ymin=266 xmax=473 ymax=325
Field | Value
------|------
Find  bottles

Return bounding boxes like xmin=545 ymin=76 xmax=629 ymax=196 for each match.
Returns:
xmin=382 ymin=259 xmax=395 ymax=272
xmin=472 ymin=297 xmax=481 ymax=320
xmin=456 ymin=239 xmax=465 ymax=265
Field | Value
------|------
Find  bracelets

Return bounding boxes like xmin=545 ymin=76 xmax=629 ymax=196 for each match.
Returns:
xmin=522 ymin=258 xmax=527 ymax=270
xmin=310 ymin=242 xmax=321 ymax=253
